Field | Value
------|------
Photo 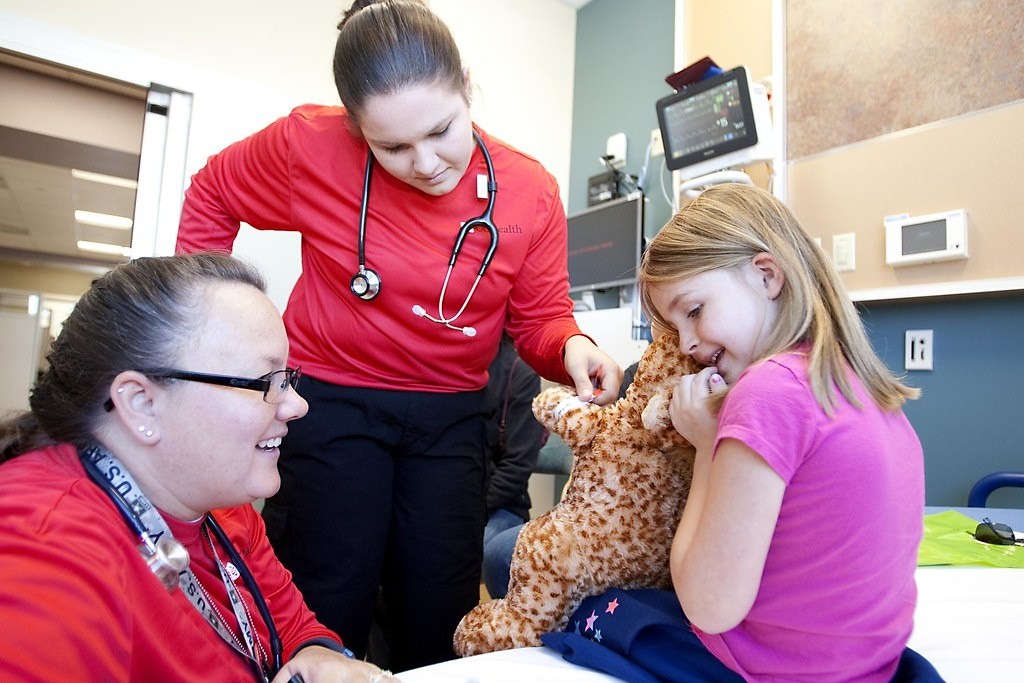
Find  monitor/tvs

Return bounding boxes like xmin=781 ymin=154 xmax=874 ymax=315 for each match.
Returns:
xmin=656 ymin=64 xmax=760 ymax=172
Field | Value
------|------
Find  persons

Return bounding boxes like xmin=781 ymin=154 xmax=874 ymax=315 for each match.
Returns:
xmin=479 ymin=310 xmax=670 ymax=600
xmin=1 ymin=248 xmax=398 ymax=683
xmin=175 ymin=0 xmax=624 ymax=676
xmin=538 ymin=181 xmax=924 ymax=683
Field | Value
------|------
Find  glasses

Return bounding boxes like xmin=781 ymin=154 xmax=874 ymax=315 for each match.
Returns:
xmin=972 ymin=517 xmax=1024 ymax=548
xmin=102 ymin=365 xmax=301 ymax=413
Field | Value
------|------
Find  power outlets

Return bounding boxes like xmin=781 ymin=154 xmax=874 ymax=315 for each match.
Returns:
xmin=905 ymin=329 xmax=934 ymax=371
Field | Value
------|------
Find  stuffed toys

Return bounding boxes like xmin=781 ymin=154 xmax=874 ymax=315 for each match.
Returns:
xmin=452 ymin=314 xmax=711 ymax=657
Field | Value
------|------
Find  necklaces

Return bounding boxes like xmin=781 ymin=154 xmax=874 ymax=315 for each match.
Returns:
xmin=190 ymin=526 xmax=270 ymax=683
